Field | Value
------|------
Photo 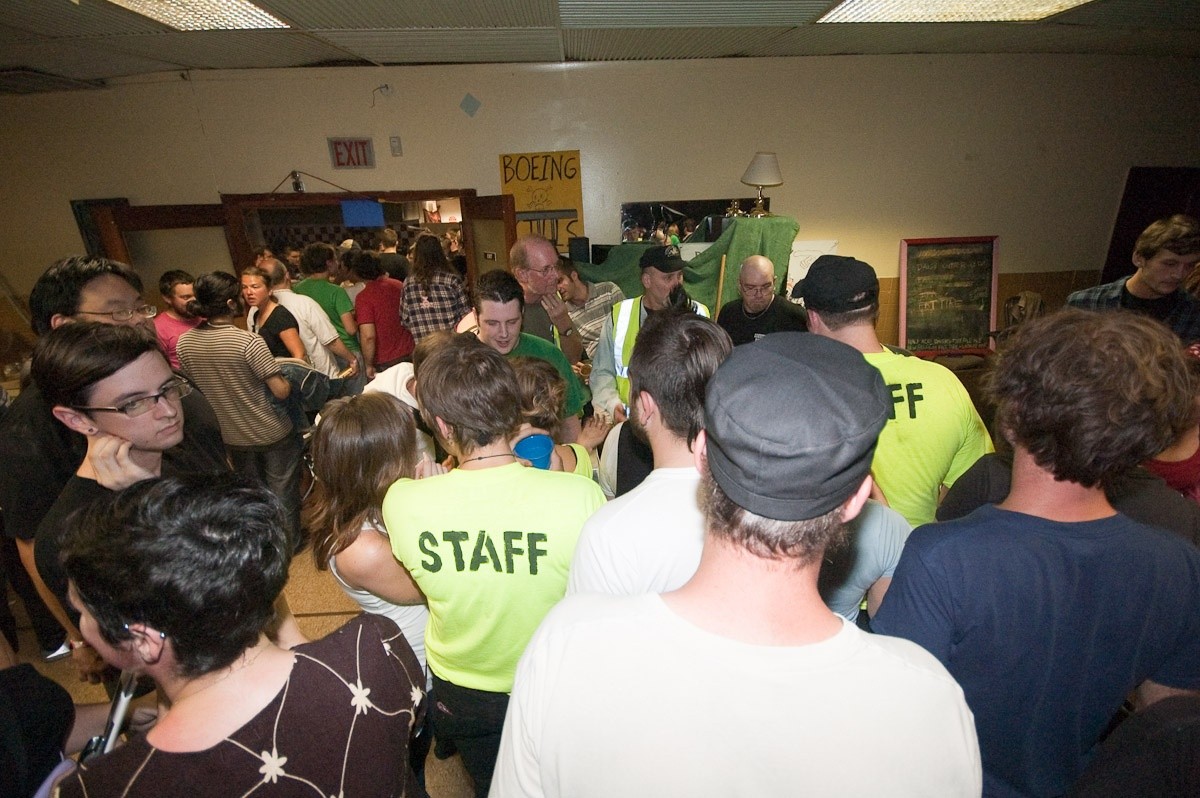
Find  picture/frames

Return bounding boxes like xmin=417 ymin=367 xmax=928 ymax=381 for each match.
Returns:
xmin=898 ymin=235 xmax=999 ymax=357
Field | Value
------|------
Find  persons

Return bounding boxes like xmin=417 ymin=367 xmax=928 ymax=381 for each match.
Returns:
xmin=0 ymin=218 xmax=1200 ymax=798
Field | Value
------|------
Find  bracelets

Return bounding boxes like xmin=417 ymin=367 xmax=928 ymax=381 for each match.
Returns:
xmin=348 ymin=357 xmax=359 ymax=366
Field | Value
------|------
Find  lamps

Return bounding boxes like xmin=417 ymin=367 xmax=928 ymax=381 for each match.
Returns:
xmin=740 ymin=152 xmax=783 ymax=217
xmin=651 ymin=204 xmax=664 ymax=236
xmin=659 ymin=204 xmax=687 ymax=243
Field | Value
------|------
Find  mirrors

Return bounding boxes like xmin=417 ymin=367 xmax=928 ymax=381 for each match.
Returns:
xmin=620 ymin=197 xmax=770 ymax=243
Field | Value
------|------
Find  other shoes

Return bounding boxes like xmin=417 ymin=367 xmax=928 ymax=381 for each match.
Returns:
xmin=45 ymin=634 xmax=75 ymax=663
xmin=291 ymin=525 xmax=310 ymax=556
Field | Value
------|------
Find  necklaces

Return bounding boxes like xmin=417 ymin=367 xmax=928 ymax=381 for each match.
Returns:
xmin=742 ymin=294 xmax=774 ymax=320
xmin=168 ymin=643 xmax=271 ymax=705
xmin=462 ymin=454 xmax=516 ymax=466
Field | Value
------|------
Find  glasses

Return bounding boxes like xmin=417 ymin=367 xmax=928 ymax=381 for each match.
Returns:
xmin=73 ymin=304 xmax=157 ymax=322
xmin=524 ymin=258 xmax=563 ymax=277
xmin=740 ymin=278 xmax=774 ymax=296
xmin=67 ymin=372 xmax=193 ymax=418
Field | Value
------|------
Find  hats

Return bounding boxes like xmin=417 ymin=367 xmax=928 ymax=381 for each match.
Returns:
xmin=336 ymin=238 xmax=362 ymax=254
xmin=640 ymin=245 xmax=687 ymax=273
xmin=704 ymin=331 xmax=890 ymax=521
xmin=792 ymin=255 xmax=879 ymax=313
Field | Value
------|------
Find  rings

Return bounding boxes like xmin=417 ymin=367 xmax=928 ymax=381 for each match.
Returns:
xmin=551 ymin=306 xmax=555 ymax=310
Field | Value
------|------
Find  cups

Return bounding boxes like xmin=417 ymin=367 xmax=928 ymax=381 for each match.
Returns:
xmin=514 ymin=435 xmax=554 ymax=470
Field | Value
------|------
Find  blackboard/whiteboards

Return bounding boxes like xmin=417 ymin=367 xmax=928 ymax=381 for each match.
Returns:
xmin=899 ymin=235 xmax=998 ymax=357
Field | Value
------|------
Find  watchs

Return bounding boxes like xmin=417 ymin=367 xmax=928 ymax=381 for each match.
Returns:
xmin=559 ymin=322 xmax=574 ymax=337
